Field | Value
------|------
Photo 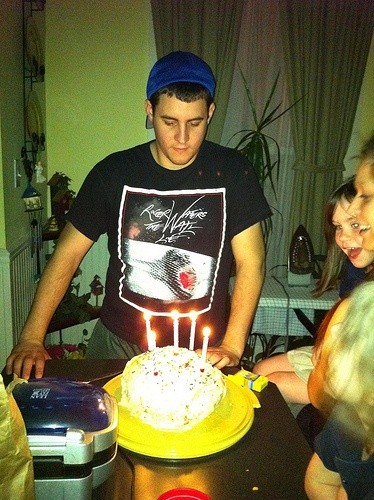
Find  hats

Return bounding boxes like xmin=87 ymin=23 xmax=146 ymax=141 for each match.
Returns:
xmin=145 ymin=51 xmax=216 ymax=130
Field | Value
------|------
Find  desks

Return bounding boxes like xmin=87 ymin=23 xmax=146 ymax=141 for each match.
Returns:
xmin=227 ymin=276 xmax=342 ymax=365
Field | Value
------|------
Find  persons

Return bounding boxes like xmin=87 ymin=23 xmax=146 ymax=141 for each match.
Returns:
xmin=252 ymin=132 xmax=374 ymax=500
xmin=6 ymin=50 xmax=272 ymax=381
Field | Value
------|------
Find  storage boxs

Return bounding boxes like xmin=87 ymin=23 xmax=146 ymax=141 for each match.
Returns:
xmin=10 ymin=376 xmax=118 ymax=500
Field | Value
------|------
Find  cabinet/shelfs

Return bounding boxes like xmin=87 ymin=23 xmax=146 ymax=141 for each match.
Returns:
xmin=37 ymin=222 xmax=103 ymax=361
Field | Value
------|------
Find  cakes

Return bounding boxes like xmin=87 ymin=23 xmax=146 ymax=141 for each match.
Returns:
xmin=119 ymin=345 xmax=226 ymax=429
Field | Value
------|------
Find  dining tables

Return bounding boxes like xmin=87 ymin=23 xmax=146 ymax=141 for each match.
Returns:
xmin=0 ymin=357 xmax=313 ymax=500
